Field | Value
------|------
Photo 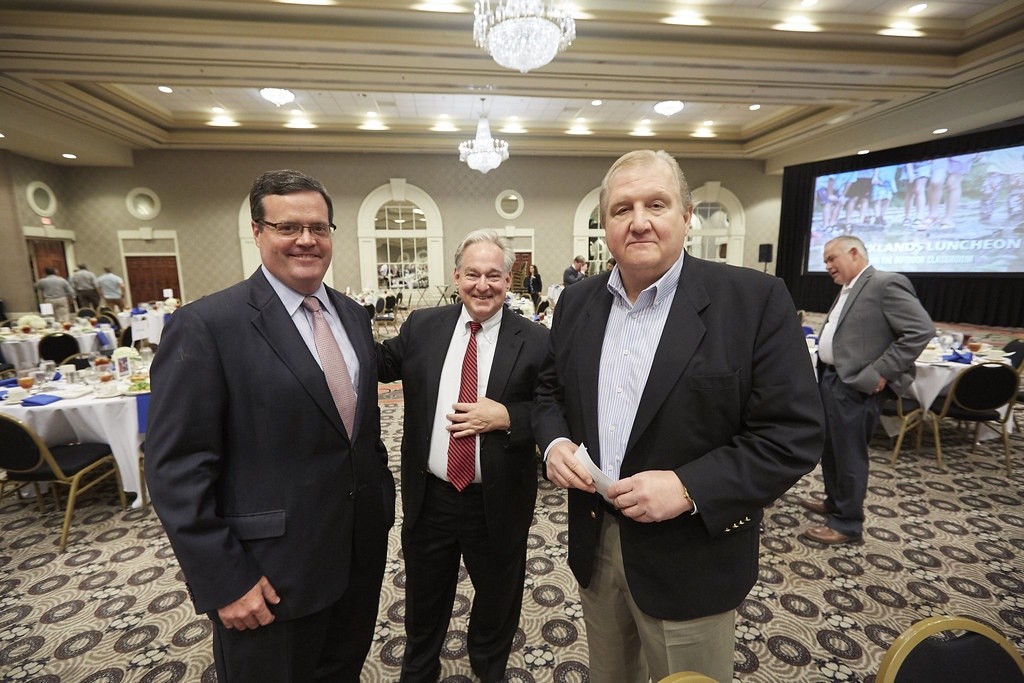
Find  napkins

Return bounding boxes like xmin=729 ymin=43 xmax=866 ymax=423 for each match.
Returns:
xmin=22 ymin=394 xmax=62 ymax=406
xmin=54 ymin=373 xmax=60 ymax=380
xmin=0 ymin=378 xmax=20 ymax=388
xmin=137 ymin=394 xmax=152 ymax=433
xmin=942 ymin=348 xmax=973 ymax=364
xmin=96 ymin=323 xmax=115 ymax=329
xmin=131 ymin=307 xmax=147 ymax=314
xmin=97 ymin=333 xmax=109 ymax=344
xmin=802 ymin=326 xmax=812 ymax=338
xmin=164 ymin=314 xmax=170 ymax=321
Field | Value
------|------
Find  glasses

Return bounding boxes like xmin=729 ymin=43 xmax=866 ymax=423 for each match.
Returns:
xmin=259 ymin=219 xmax=337 ymax=235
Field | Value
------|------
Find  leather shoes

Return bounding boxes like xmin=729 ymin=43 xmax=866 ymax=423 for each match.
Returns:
xmin=800 ymin=498 xmax=824 ymax=516
xmin=805 ymin=525 xmax=862 ymax=544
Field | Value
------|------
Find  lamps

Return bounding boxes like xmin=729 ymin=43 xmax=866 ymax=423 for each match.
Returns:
xmin=472 ymin=0 xmax=576 ymax=76
xmin=457 ymin=98 xmax=510 ymax=175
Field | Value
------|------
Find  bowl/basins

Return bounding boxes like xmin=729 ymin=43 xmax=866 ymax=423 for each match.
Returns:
xmin=0 ymin=327 xmax=11 ymax=333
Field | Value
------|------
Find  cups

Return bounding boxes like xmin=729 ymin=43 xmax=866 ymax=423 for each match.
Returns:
xmin=2 ymin=387 xmax=27 ymax=403
xmin=979 ymin=344 xmax=993 ymax=351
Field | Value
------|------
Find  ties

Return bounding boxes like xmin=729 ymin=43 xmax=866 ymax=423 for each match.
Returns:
xmin=446 ymin=321 xmax=482 ymax=494
xmin=303 ymin=295 xmax=357 ymax=440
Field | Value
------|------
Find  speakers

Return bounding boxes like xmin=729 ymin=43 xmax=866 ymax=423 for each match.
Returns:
xmin=758 ymin=244 xmax=773 ymax=262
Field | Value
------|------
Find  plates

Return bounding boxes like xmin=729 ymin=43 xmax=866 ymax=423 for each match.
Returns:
xmin=97 ymin=383 xmax=129 ymax=398
xmin=916 ymin=357 xmax=944 ymax=363
xmin=124 ymin=384 xmax=152 ymax=396
xmin=4 ymin=397 xmax=31 ymax=405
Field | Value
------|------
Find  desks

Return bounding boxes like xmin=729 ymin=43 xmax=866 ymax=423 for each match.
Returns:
xmin=435 ymin=284 xmax=451 ymax=306
xmin=511 ymin=301 xmax=535 ymax=319
xmin=540 ymin=315 xmax=553 ymax=330
xmin=808 ymin=340 xmax=1013 ymax=441
xmin=0 ymin=359 xmax=150 ymax=507
xmin=117 ymin=309 xmax=173 ymax=346
xmin=415 ymin=288 xmax=426 ymax=308
xmin=0 ymin=332 xmax=117 ymax=373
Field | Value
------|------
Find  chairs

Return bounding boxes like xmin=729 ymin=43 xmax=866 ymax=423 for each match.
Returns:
xmin=0 ymin=307 xmax=152 ymax=554
xmin=876 ymin=615 xmax=1024 ymax=683
xmin=874 ymin=338 xmax=1024 ymax=478
xmin=364 ymin=293 xmax=414 ymax=341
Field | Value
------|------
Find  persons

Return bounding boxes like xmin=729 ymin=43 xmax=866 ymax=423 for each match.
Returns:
xmin=33 ymin=262 xmax=102 ymax=324
xmin=564 ymin=256 xmax=588 ymax=288
xmin=814 ymin=146 xmax=1024 ymax=238
xmin=527 ymin=264 xmax=542 ymax=307
xmin=370 ymin=228 xmax=552 ymax=683
xmin=98 ymin=265 xmax=126 ymax=312
xmin=532 ymin=148 xmax=827 ymax=683
xmin=803 ymin=236 xmax=936 ymax=542
xmin=606 ymin=258 xmax=616 ymax=272
xmin=142 ymin=170 xmax=396 ymax=683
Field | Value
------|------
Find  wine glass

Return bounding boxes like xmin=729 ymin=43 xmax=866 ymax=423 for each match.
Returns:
xmin=939 ymin=330 xmax=981 ymax=359
xmin=13 ymin=316 xmax=99 ymax=337
xmin=17 ymin=347 xmax=155 ymax=398
